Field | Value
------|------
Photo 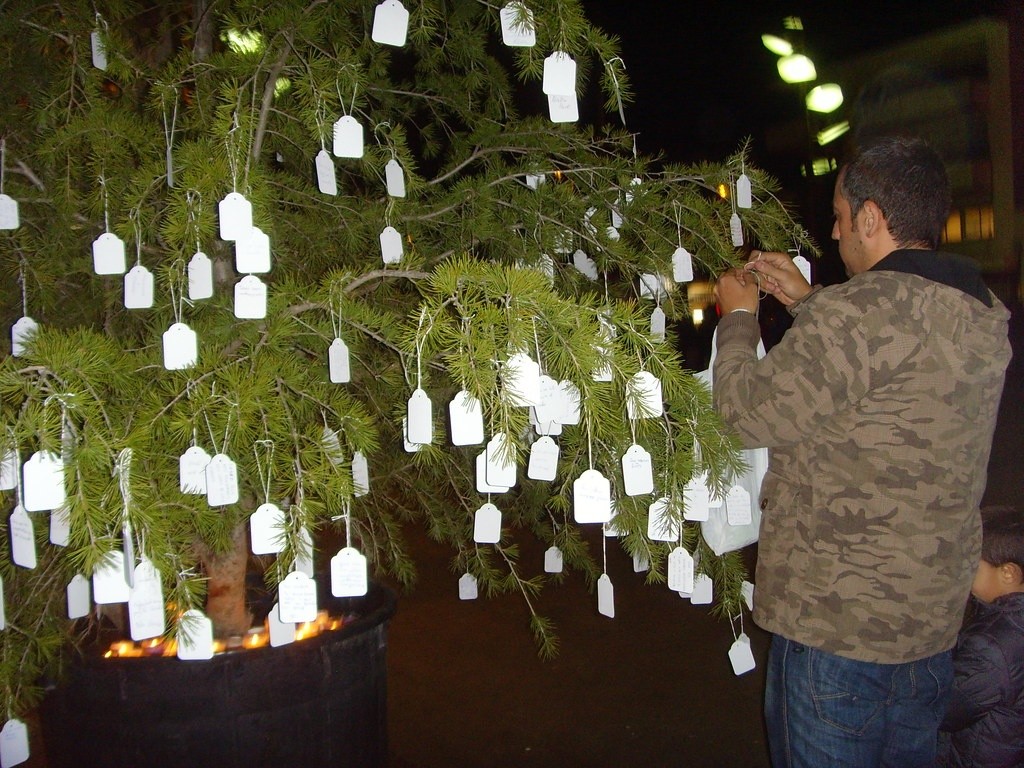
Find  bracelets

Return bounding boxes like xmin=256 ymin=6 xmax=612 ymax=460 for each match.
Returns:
xmin=731 ymin=308 xmax=750 ymax=314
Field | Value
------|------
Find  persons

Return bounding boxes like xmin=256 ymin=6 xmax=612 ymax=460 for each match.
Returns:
xmin=711 ymin=134 xmax=1013 ymax=767
xmin=940 ymin=503 xmax=1024 ymax=768
